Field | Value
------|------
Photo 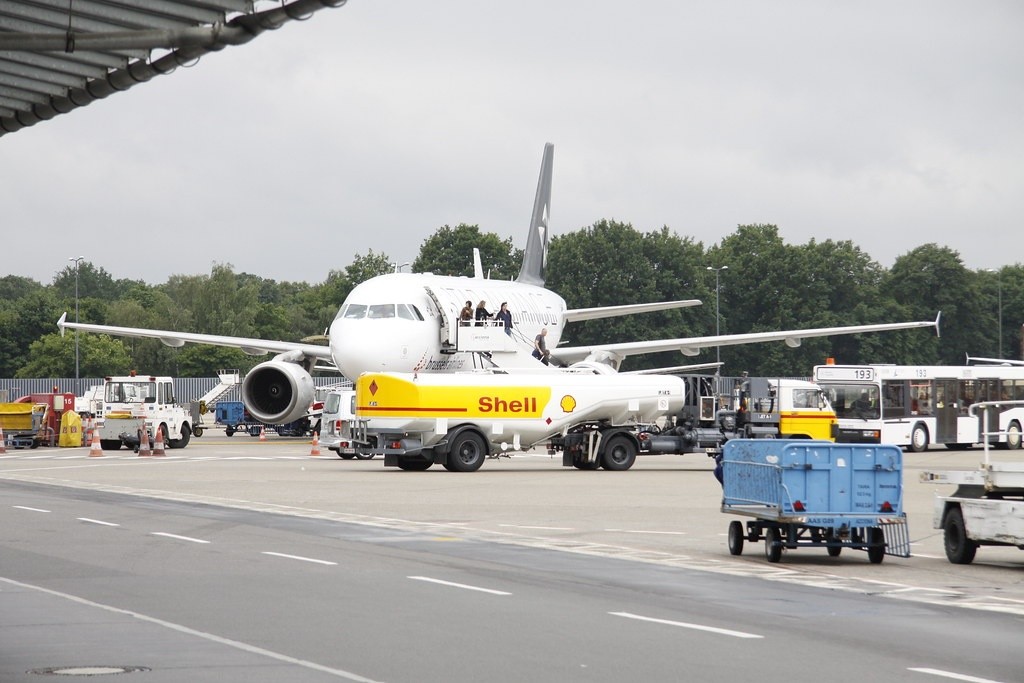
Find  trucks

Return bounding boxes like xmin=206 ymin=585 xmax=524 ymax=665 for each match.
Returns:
xmin=923 ymin=463 xmax=1024 ymax=563
xmin=703 ymin=437 xmax=912 ymax=562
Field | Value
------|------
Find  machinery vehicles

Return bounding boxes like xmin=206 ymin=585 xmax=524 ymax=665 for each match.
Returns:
xmin=0 ymin=370 xmax=194 ymax=450
xmin=542 ymin=369 xmax=839 ymax=472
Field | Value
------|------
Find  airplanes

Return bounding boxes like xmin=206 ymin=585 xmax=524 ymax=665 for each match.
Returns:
xmin=54 ymin=141 xmax=944 ymax=473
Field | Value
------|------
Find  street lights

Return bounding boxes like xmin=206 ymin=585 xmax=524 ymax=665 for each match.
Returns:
xmin=706 ymin=265 xmax=728 ymax=374
xmin=66 ymin=256 xmax=85 ymax=376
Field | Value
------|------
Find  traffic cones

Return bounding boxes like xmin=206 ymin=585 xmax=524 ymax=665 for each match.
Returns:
xmin=258 ymin=425 xmax=267 ymax=443
xmin=138 ymin=422 xmax=152 ymax=457
xmin=0 ymin=428 xmax=7 ymax=454
xmin=307 ymin=431 xmax=322 ymax=457
xmin=88 ymin=422 xmax=105 ymax=456
xmin=81 ymin=414 xmax=93 ymax=447
xmin=152 ymin=427 xmax=167 ymax=457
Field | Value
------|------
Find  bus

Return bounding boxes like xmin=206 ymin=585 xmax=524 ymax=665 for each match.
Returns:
xmin=811 ymin=356 xmax=1024 ymax=453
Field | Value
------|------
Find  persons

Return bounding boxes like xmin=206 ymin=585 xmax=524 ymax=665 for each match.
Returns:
xmin=918 ymin=390 xmax=928 ymax=408
xmin=702 ymin=403 xmax=713 ymax=417
xmin=872 ymin=389 xmax=889 ymax=408
xmin=475 ymin=300 xmax=494 ymax=327
xmin=459 ymin=301 xmax=474 ymax=327
xmin=850 ymin=393 xmax=872 ymax=409
xmin=494 ymin=302 xmax=513 ymax=337
xmin=982 ymin=385 xmax=1024 ymax=401
xmin=534 ymin=328 xmax=549 ymax=366
